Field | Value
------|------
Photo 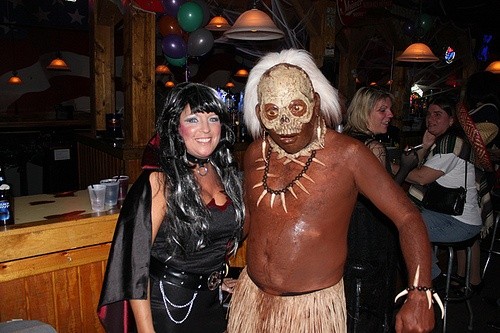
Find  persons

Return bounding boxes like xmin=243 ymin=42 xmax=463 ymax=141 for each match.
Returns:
xmin=225 ymin=48 xmax=436 ymax=332
xmin=96 ymin=82 xmax=245 ymax=333
xmin=341 ymin=84 xmax=419 ymax=265
xmin=387 ymin=71 xmax=500 ymax=297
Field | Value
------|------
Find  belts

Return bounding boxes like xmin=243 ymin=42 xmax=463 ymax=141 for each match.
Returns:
xmin=149 ymin=256 xmax=226 ymax=291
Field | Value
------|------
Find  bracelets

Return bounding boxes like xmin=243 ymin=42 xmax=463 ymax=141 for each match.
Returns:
xmin=394 ymin=265 xmax=445 ymax=320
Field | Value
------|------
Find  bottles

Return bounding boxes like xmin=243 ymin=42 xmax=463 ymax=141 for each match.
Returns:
xmin=106 ymin=112 xmax=123 ymax=130
xmin=215 ymin=85 xmax=244 ymax=126
xmin=0 ymin=166 xmax=15 ymax=225
xmin=402 ymin=132 xmax=412 ymax=156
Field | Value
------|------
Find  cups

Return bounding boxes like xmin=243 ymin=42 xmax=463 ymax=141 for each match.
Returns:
xmin=100 ymin=179 xmax=120 ymax=205
xmin=115 ymin=137 xmax=126 ymax=146
xmin=113 ymin=176 xmax=130 ymax=201
xmin=88 ymin=185 xmax=106 ymax=211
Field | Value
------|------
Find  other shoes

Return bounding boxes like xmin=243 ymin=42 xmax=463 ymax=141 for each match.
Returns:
xmin=454 ymin=281 xmax=482 ymax=297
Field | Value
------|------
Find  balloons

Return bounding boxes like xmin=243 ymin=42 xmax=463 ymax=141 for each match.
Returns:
xmin=134 ymin=0 xmax=213 ymax=77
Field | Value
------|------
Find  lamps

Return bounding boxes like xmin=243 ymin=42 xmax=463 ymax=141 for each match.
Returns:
xmin=223 ymin=0 xmax=284 ymax=40
xmin=205 ymin=0 xmax=231 ymax=32
xmin=395 ymin=0 xmax=441 ymax=63
xmin=47 ymin=57 xmax=70 ymax=70
xmin=10 ymin=52 xmax=22 ymax=84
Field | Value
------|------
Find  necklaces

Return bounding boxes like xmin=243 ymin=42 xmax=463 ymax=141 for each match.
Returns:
xmin=252 ymin=143 xmax=326 ymax=215
xmin=186 ymin=151 xmax=211 ymax=176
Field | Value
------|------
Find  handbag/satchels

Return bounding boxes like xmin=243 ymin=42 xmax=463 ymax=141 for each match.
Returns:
xmin=407 ymin=159 xmax=467 ymax=216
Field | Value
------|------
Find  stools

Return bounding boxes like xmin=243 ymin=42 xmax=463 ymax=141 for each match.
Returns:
xmin=349 ymin=187 xmax=500 ymax=333
